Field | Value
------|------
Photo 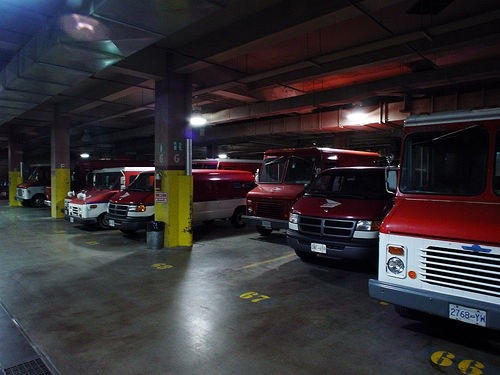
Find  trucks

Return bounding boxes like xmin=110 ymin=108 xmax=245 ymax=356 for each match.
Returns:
xmin=46 ymin=161 xmax=135 ymax=224
xmin=68 ymin=167 xmax=156 ymax=232
xmin=103 ymin=169 xmax=255 ymax=235
xmin=14 ymin=166 xmax=49 ymax=207
xmin=245 ymin=146 xmax=382 ymax=237
xmin=192 ymin=158 xmax=262 ymax=174
xmin=368 ymin=108 xmax=500 ymax=332
xmin=283 ymin=166 xmax=383 ymax=271
